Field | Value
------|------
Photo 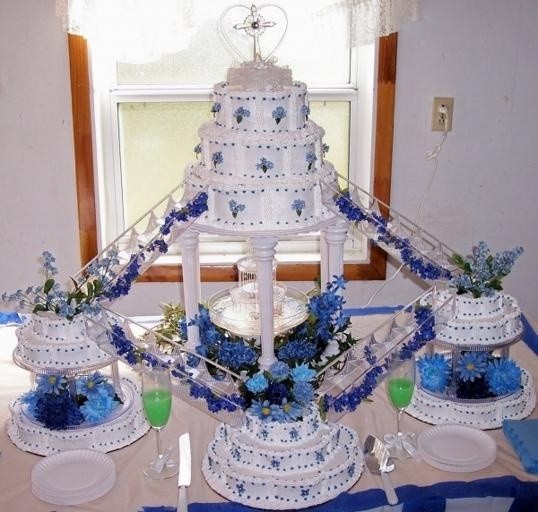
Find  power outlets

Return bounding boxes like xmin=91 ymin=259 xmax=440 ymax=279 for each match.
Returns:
xmin=431 ymin=96 xmax=454 ymax=134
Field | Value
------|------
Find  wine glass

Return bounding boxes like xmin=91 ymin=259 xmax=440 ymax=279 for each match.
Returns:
xmin=383 ymin=351 xmax=416 ymax=445
xmin=141 ymin=367 xmax=179 ymax=479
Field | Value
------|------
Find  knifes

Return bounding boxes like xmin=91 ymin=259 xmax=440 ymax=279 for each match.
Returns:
xmin=176 ymin=433 xmax=191 ymax=512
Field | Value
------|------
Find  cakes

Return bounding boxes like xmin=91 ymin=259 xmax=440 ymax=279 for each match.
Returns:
xmin=11 ymin=306 xmax=137 ymax=451
xmin=207 ymin=400 xmax=362 ymax=502
xmin=408 ymin=283 xmax=533 ymax=426
xmin=182 ymin=81 xmax=341 ymax=231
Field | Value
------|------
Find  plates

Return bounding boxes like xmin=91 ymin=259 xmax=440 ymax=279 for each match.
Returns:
xmin=30 ymin=449 xmax=116 ymax=505
xmin=417 ymin=425 xmax=498 ymax=475
